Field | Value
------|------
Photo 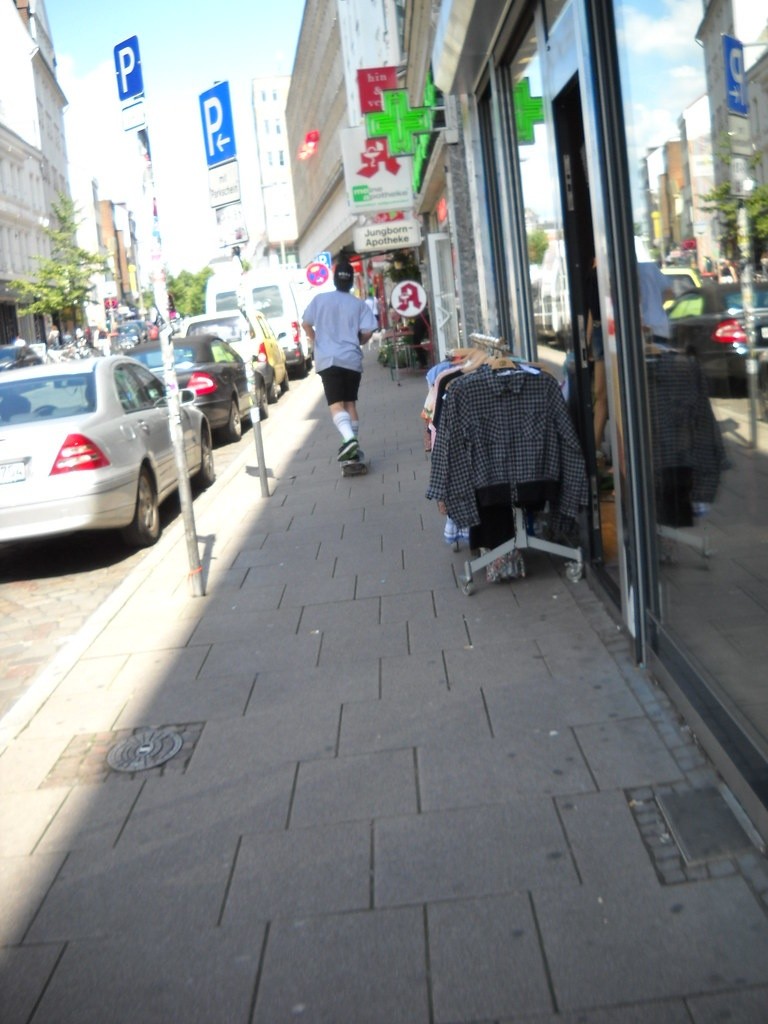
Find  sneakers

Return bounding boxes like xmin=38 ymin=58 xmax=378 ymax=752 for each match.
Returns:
xmin=337 ymin=439 xmax=358 ymax=462
xmin=347 ymin=451 xmax=364 ymax=464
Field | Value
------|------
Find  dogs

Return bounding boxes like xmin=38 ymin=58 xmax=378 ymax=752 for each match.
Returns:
xmin=368 ymin=329 xmax=386 ymax=349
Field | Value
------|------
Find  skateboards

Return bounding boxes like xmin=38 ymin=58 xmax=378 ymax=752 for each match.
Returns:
xmin=338 ymin=448 xmax=369 ymax=478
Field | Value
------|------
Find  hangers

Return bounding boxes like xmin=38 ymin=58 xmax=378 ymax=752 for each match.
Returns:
xmin=439 ymin=345 xmax=551 ymax=394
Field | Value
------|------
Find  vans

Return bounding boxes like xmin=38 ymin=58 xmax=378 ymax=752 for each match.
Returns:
xmin=206 ymin=264 xmax=313 ymax=379
xmin=181 ymin=308 xmax=289 ymax=403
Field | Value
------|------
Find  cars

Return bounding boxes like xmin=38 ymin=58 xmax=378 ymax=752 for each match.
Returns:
xmin=536 ymin=235 xmax=768 ymax=399
xmin=124 ymin=335 xmax=268 ymax=442
xmin=1 ymin=356 xmax=215 ymax=547
xmin=0 ymin=298 xmax=181 ymax=372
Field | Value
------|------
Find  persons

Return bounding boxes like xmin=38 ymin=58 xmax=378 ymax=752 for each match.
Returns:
xmin=11 ymin=318 xmax=172 ymax=366
xmin=717 ymin=259 xmax=738 ymax=285
xmin=302 ymin=262 xmax=379 ymax=463
xmin=637 ymin=240 xmax=677 ymax=346
xmin=365 ymin=291 xmax=380 ymax=331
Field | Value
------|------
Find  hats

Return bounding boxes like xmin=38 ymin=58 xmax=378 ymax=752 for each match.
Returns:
xmin=334 ymin=262 xmax=354 ymax=287
